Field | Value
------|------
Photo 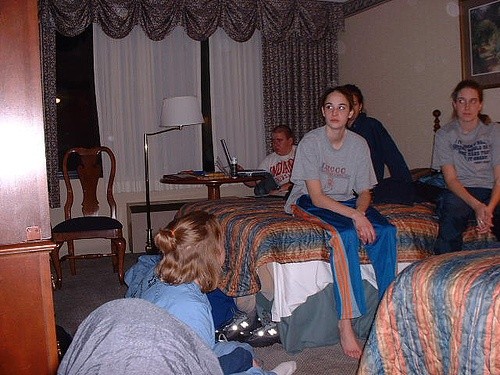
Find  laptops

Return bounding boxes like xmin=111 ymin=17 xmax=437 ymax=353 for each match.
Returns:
xmin=220 ymin=139 xmax=267 ymax=176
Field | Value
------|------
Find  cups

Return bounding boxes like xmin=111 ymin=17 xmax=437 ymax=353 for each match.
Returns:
xmin=231 ymin=157 xmax=238 ymax=179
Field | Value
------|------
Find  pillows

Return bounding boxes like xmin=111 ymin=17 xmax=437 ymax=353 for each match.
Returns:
xmin=410 ymin=168 xmax=438 ymax=181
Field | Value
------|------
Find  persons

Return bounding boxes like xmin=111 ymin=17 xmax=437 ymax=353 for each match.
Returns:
xmin=342 ymin=84 xmax=415 ymax=207
xmin=284 ymin=87 xmax=398 ymax=358
xmin=236 ymin=124 xmax=299 ymax=198
xmin=123 ymin=210 xmax=297 ymax=375
xmin=431 ymin=79 xmax=500 ymax=256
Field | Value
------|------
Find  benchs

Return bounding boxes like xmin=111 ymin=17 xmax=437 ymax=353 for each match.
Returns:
xmin=127 ymin=196 xmax=240 ymax=254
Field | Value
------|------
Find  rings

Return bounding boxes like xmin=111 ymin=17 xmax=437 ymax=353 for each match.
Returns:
xmin=479 ymin=220 xmax=483 ymax=224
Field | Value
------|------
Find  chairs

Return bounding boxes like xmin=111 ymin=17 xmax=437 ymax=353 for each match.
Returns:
xmin=51 ymin=147 xmax=126 ymax=289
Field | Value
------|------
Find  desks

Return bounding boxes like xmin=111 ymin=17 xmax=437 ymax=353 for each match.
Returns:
xmin=160 ymin=169 xmax=268 ymax=200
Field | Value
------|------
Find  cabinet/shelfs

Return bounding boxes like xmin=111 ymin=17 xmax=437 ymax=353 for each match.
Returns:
xmin=0 ymin=0 xmax=59 ymax=375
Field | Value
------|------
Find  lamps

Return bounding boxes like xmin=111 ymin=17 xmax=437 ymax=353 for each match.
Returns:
xmin=144 ymin=95 xmax=205 ymax=255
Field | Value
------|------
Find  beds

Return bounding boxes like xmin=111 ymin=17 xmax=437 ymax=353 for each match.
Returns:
xmin=356 ymin=248 xmax=500 ymax=375
xmin=174 ymin=173 xmax=500 ymax=353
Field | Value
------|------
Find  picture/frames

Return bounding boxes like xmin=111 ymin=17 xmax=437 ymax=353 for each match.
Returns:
xmin=458 ymin=0 xmax=500 ymax=90
